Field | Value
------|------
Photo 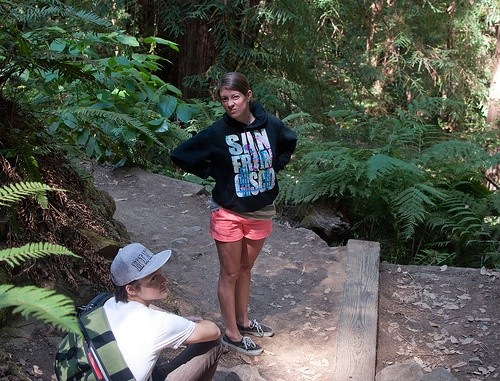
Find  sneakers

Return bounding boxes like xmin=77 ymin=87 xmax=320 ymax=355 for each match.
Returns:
xmin=221 ymin=332 xmax=264 ymax=357
xmin=238 ymin=321 xmax=275 ymax=338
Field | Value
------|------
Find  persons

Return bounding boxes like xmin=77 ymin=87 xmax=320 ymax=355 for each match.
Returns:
xmin=172 ymin=73 xmax=297 ymax=355
xmin=81 ymin=243 xmax=221 ymax=381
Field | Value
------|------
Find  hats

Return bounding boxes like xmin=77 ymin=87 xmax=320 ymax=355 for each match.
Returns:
xmin=109 ymin=242 xmax=173 ymax=285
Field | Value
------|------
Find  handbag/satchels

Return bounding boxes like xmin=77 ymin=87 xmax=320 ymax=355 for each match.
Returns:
xmin=79 ymin=290 xmax=114 ymax=316
